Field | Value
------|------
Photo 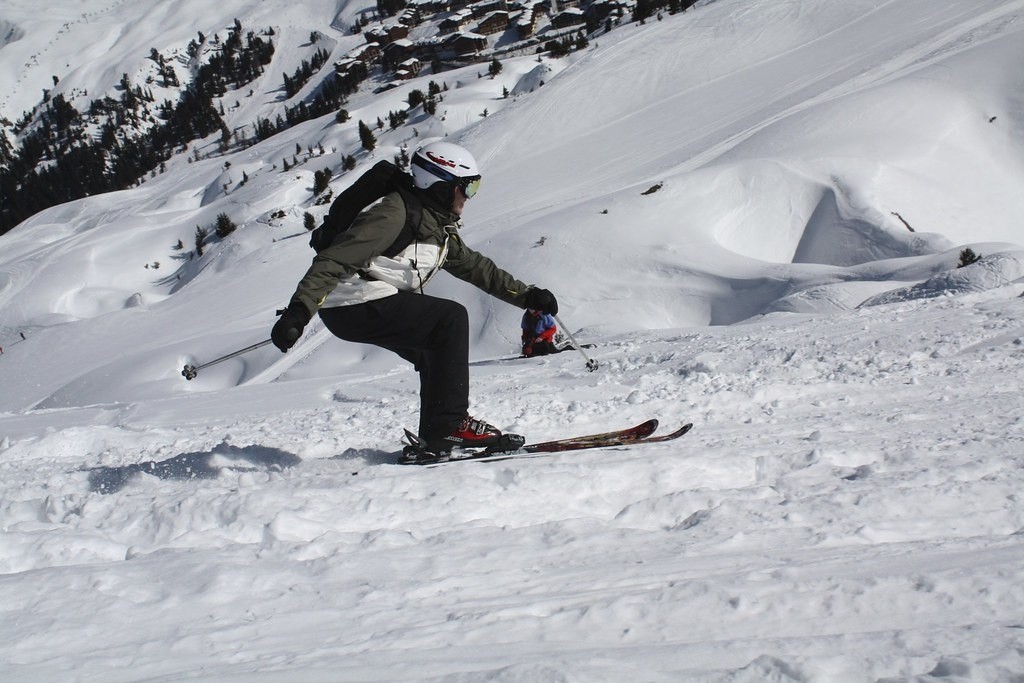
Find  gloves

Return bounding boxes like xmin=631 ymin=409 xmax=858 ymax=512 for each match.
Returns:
xmin=523 ymin=286 xmax=558 ymax=317
xmin=271 ymin=307 xmax=308 ymax=353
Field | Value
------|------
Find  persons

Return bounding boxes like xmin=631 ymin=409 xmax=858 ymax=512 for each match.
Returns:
xmin=520 ymin=307 xmax=557 ymax=356
xmin=270 ymin=139 xmax=558 ymax=450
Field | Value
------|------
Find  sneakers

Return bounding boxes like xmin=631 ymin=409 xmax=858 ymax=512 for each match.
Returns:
xmin=418 ymin=416 xmax=503 ymax=451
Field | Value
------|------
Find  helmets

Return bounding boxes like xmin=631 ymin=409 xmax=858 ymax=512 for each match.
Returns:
xmin=411 ymin=142 xmax=480 ymax=190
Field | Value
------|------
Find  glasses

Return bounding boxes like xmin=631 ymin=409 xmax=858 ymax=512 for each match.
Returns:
xmin=461 ymin=177 xmax=480 ymax=197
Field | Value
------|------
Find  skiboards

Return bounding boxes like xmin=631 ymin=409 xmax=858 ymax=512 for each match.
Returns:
xmin=396 ymin=418 xmax=694 ymax=469
xmin=519 ymin=344 xmax=597 ymax=359
